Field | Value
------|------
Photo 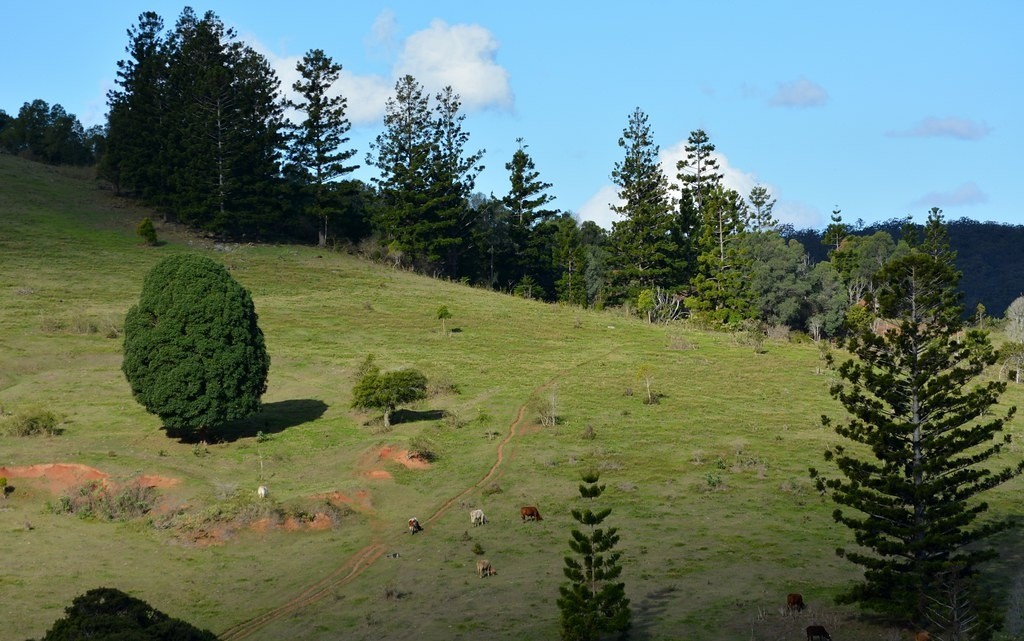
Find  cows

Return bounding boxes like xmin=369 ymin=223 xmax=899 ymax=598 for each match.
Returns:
xmin=258 ymin=486 xmax=268 ymax=498
xmin=477 ymin=560 xmax=496 ymax=578
xmin=915 ymin=631 xmax=933 ymax=641
xmin=520 ymin=506 xmax=543 ymax=522
xmin=806 ymin=625 xmax=831 ymax=641
xmin=408 ymin=518 xmax=423 ymax=536
xmin=471 ymin=509 xmax=490 ymax=527
xmin=787 ymin=593 xmax=808 ymax=612
